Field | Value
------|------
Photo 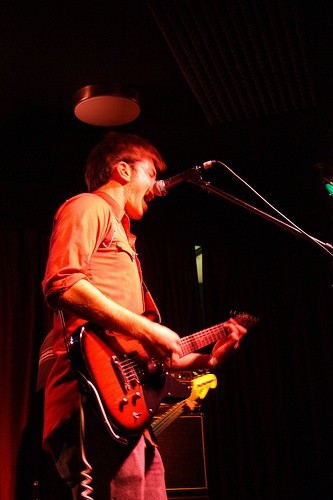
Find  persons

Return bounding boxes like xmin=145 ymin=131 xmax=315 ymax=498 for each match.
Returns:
xmin=34 ymin=130 xmax=249 ymax=500
xmin=41 ymin=135 xmax=85 ymax=175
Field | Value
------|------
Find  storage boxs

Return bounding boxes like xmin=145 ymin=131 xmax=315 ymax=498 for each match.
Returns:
xmin=149 ymin=400 xmax=209 ymax=494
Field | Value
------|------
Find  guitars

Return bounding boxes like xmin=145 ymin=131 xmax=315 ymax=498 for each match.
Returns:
xmin=66 ymin=309 xmax=257 ymax=437
xmin=149 ymin=369 xmax=218 ymax=439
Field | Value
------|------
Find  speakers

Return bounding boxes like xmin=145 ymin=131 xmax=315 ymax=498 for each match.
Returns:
xmin=150 ymin=411 xmax=208 ymax=494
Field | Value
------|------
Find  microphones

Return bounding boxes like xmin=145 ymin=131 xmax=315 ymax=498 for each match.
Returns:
xmin=152 ymin=160 xmax=212 ymax=197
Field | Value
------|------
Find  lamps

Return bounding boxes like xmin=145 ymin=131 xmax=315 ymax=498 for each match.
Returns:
xmin=73 ymin=83 xmax=142 ymax=128
